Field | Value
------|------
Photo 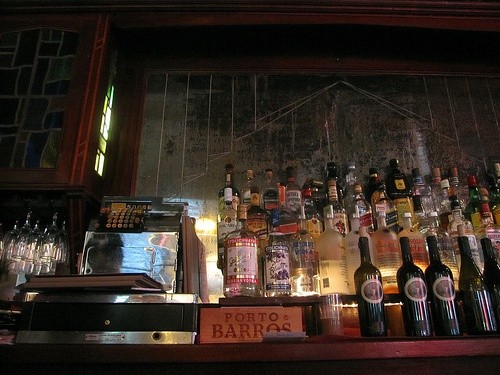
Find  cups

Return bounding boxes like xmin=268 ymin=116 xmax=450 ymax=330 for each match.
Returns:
xmin=317 ymin=294 xmax=345 ymax=337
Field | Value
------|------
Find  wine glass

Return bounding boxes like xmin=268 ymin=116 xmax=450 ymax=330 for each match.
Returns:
xmin=0 ymin=194 xmax=69 ymax=276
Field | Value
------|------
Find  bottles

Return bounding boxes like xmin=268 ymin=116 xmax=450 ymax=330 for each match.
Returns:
xmin=457 ymin=235 xmax=498 ymax=335
xmin=246 ymin=186 xmax=269 ymax=261
xmin=224 ymin=204 xmax=261 ymax=296
xmin=425 ymin=236 xmax=465 ymax=336
xmin=480 ymin=237 xmax=500 ymax=329
xmin=262 ymin=159 xmax=500 ymax=297
xmin=217 ymin=168 xmax=241 ymax=211
xmin=397 ymin=236 xmax=433 ymax=337
xmin=217 ymin=188 xmax=237 ymax=269
xmin=354 ymin=236 xmax=387 ymax=336
xmin=242 ymin=169 xmax=261 ymax=206
